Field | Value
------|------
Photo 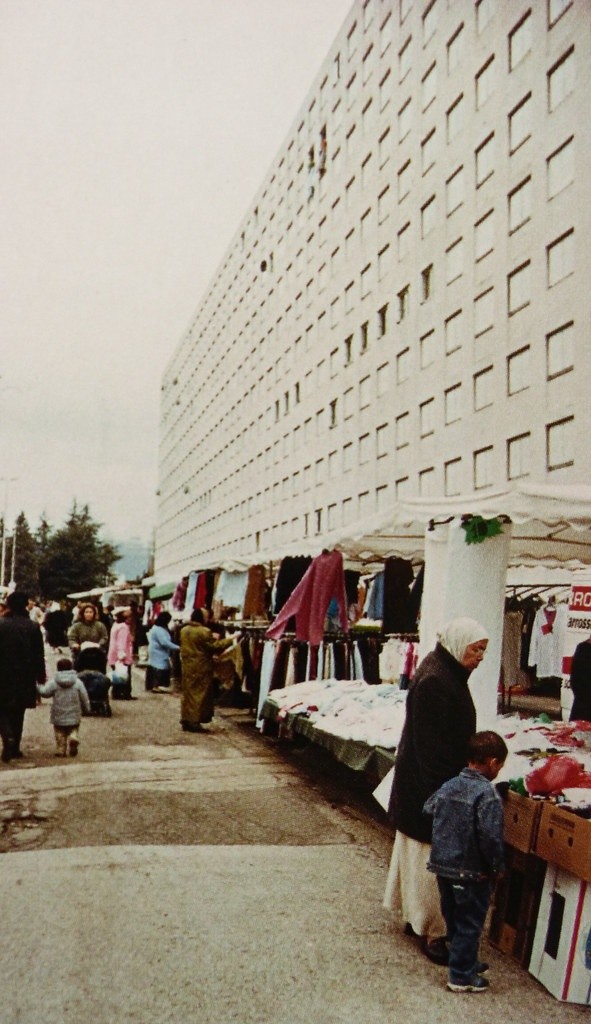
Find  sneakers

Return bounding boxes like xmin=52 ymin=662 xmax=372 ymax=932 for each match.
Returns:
xmin=447 ymin=961 xmax=489 ymax=992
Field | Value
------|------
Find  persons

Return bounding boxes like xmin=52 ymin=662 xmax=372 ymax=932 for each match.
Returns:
xmin=423 ymin=731 xmax=508 ymax=991
xmin=179 ymin=608 xmax=239 ymax=732
xmin=144 ymin=612 xmax=180 ymax=692
xmin=0 ymin=591 xmax=134 ymax=761
xmin=381 ymin=618 xmax=489 ymax=969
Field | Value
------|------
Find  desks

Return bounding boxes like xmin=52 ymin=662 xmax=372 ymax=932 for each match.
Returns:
xmin=259 ymin=699 xmax=396 ymax=813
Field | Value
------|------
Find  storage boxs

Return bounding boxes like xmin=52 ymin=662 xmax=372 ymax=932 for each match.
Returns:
xmin=496 ymin=787 xmax=590 ymax=1006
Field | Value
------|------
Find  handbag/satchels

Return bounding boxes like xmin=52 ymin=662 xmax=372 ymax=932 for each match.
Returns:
xmin=110 ymin=660 xmax=129 ymax=683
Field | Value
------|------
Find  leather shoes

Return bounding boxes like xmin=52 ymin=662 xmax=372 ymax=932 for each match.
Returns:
xmin=421 ymin=937 xmax=449 ymax=965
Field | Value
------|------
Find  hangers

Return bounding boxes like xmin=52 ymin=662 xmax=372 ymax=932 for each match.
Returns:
xmin=509 ymin=591 xmax=571 ymax=613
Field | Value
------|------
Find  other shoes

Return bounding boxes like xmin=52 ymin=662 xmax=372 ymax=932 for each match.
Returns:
xmin=55 ymin=752 xmax=67 ymax=757
xmin=151 ymin=689 xmax=167 ymax=693
xmin=112 ymin=695 xmax=138 ymax=700
xmin=68 ymin=740 xmax=79 ymax=757
xmin=1 ymin=747 xmax=22 ymax=762
xmin=183 ymin=721 xmax=210 ymax=732
